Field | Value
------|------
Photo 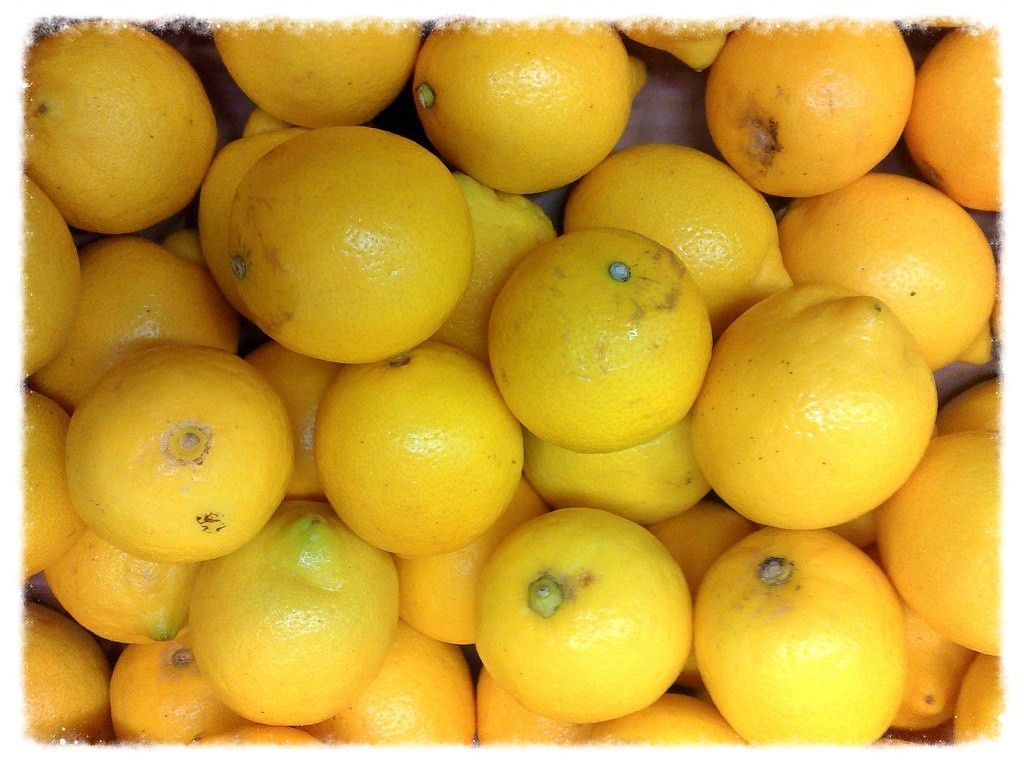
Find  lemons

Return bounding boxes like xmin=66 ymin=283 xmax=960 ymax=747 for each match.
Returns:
xmin=22 ymin=18 xmax=1002 ymax=748
